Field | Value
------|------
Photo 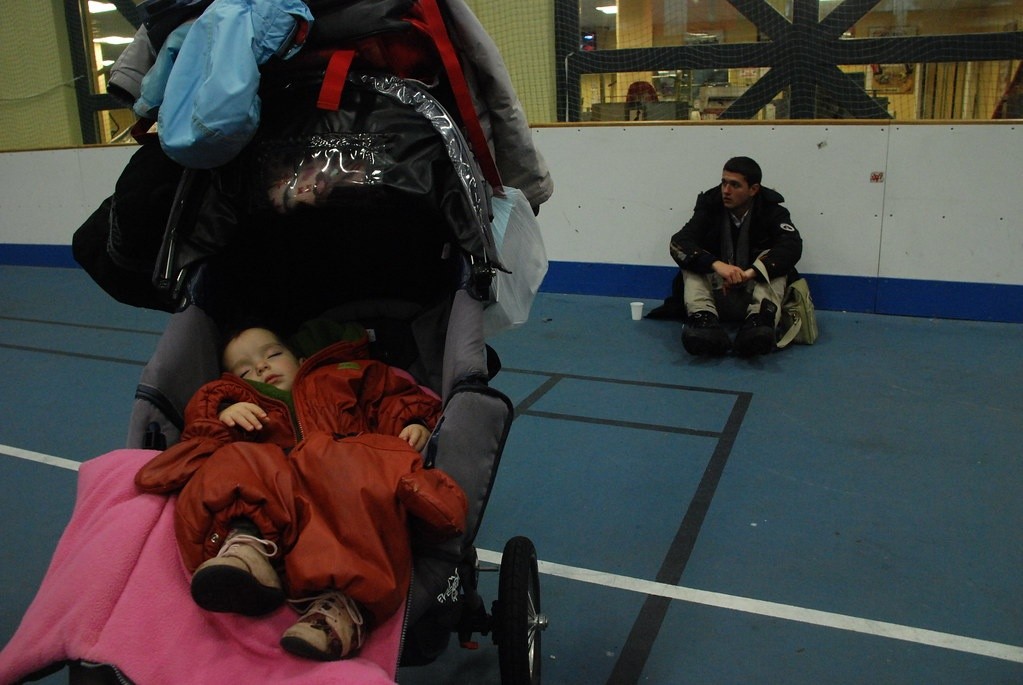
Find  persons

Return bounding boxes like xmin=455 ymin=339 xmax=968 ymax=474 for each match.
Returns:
xmin=670 ymin=156 xmax=803 ymax=361
xmin=182 ymin=323 xmax=443 ymax=658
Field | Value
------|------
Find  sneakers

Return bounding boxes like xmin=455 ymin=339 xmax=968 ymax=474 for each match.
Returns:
xmin=681 ymin=311 xmax=732 ymax=355
xmin=733 ymin=298 xmax=778 ymax=359
xmin=278 ymin=590 xmax=373 ymax=660
xmin=189 ymin=533 xmax=284 ymax=616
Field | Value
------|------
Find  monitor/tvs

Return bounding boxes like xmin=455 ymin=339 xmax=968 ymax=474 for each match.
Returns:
xmin=580 ymin=31 xmax=597 ymax=51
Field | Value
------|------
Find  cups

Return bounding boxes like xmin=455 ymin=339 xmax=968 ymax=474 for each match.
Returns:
xmin=630 ymin=302 xmax=644 ymax=320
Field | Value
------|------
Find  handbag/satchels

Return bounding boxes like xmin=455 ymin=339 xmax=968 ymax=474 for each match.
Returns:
xmin=753 ymin=278 xmax=818 ymax=348
xmin=490 ymin=186 xmax=549 ymax=325
xmin=71 ymin=142 xmax=234 ymax=313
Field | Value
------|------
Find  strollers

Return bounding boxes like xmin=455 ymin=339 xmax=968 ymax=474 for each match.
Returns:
xmin=66 ymin=51 xmax=547 ymax=685
